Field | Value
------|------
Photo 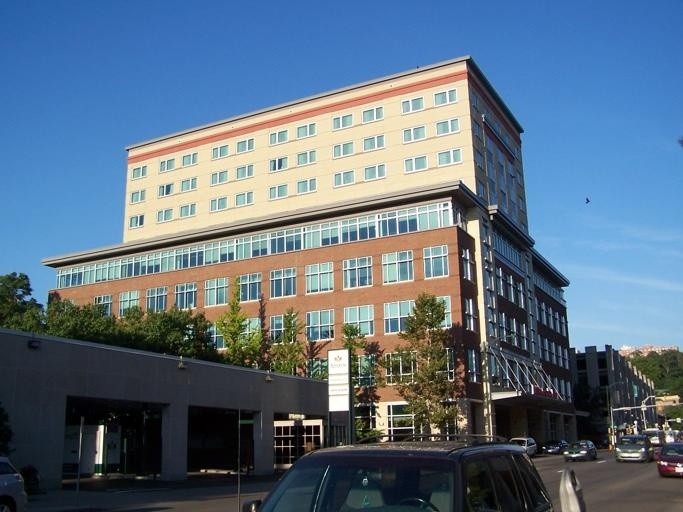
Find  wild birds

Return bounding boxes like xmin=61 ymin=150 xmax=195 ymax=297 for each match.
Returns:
xmin=585 ymin=197 xmax=590 ymax=204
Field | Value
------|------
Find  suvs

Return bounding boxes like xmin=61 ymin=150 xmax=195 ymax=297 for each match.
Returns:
xmin=239 ymin=439 xmax=555 ymax=512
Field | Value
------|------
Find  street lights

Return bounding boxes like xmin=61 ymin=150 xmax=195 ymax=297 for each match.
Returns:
xmin=641 ymin=395 xmax=655 ymax=430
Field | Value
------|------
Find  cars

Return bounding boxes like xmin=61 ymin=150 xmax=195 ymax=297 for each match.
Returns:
xmin=0 ymin=457 xmax=30 ymax=511
xmin=614 ymin=426 xmax=682 ymax=479
xmin=541 ymin=439 xmax=567 ymax=454
xmin=509 ymin=437 xmax=537 ymax=458
xmin=562 ymin=440 xmax=598 ymax=462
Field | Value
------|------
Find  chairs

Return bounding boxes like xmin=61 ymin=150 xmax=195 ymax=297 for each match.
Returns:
xmin=338 ymin=480 xmax=387 ymax=512
xmin=429 ymin=484 xmax=474 ymax=512
